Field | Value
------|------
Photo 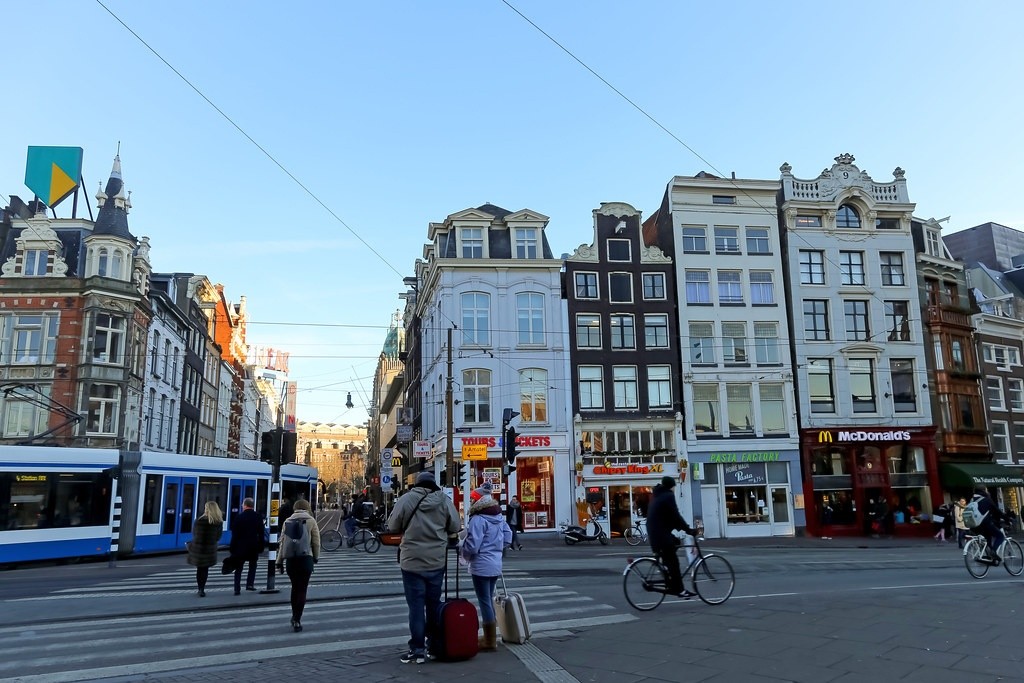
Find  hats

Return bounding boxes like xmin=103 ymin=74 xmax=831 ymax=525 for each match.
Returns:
xmin=662 ymin=476 xmax=676 ymax=489
xmin=469 ymin=482 xmax=493 ymax=500
xmin=352 ymin=494 xmax=358 ymax=499
xmin=417 ymin=472 xmax=436 ymax=483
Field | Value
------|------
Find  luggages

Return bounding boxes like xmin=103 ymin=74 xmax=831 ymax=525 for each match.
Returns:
xmin=433 ymin=546 xmax=480 ymax=661
xmin=493 ymin=573 xmax=531 ymax=645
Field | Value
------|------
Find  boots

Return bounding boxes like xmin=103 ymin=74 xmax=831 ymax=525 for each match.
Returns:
xmin=478 ymin=622 xmax=496 ymax=652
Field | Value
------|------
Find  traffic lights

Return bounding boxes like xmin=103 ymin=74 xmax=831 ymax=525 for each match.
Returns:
xmin=507 ymin=425 xmax=522 ymax=465
xmin=503 ymin=407 xmax=520 ymax=423
xmin=390 ymin=473 xmax=398 ymax=495
xmin=456 ymin=460 xmax=467 ymax=490
xmin=439 ymin=470 xmax=446 ymax=487
xmin=260 ymin=431 xmax=276 ymax=463
xmin=504 ymin=465 xmax=517 ymax=475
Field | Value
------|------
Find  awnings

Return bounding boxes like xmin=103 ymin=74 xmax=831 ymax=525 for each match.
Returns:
xmin=938 ymin=462 xmax=1024 ymax=492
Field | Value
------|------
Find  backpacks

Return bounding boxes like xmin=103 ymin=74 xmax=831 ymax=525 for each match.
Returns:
xmin=282 ymin=519 xmax=308 ymax=558
xmin=361 ymin=504 xmax=371 ymax=518
xmin=962 ymin=497 xmax=989 ymax=527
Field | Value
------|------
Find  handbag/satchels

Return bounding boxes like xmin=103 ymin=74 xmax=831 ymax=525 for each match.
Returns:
xmin=933 ymin=514 xmax=945 ymax=522
xmin=222 ymin=555 xmax=235 ymax=575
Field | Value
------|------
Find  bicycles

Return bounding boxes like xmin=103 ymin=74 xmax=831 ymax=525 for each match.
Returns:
xmin=320 ymin=512 xmax=375 ymax=552
xmin=623 ymin=521 xmax=736 ymax=611
xmin=962 ymin=520 xmax=1024 ymax=580
xmin=623 ymin=519 xmax=650 ymax=547
xmin=364 ymin=514 xmax=389 ymax=554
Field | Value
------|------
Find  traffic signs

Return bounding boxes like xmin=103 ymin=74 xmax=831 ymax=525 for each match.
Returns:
xmin=463 ymin=444 xmax=488 ymax=462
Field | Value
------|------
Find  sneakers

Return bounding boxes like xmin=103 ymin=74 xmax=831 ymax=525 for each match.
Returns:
xmin=400 ymin=650 xmax=425 ymax=664
xmin=426 ymin=651 xmax=436 ymax=660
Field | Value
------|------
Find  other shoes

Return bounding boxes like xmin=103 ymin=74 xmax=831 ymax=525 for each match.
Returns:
xmin=518 ymin=544 xmax=523 ymax=550
xmin=246 ymin=586 xmax=257 ymax=590
xmin=934 ymin=536 xmax=939 ymax=542
xmin=293 ymin=621 xmax=302 ymax=631
xmin=941 ymin=539 xmax=948 ymax=543
xmin=510 ymin=546 xmax=515 ymax=550
xmin=989 ymin=551 xmax=1002 ymax=561
xmin=198 ymin=590 xmax=205 ymax=597
xmin=678 ymin=590 xmax=698 ymax=599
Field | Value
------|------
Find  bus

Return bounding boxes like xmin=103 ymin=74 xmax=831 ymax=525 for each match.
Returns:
xmin=0 ymin=383 xmax=327 ymax=565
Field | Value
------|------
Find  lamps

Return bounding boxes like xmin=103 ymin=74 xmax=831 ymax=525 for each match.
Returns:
xmin=345 ymin=392 xmax=354 ymax=409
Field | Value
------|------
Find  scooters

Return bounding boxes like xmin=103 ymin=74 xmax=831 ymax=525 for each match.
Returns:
xmin=560 ymin=507 xmax=609 ymax=547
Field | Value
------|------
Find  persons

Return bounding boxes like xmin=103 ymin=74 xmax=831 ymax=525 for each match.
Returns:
xmin=645 ymin=476 xmax=705 ymax=595
xmin=187 ymin=501 xmax=223 ymax=597
xmin=970 ymin=485 xmax=1019 ymax=561
xmin=384 ymin=473 xmax=459 ymax=664
xmin=229 ymin=497 xmax=263 ymax=596
xmin=277 ymin=498 xmax=321 ymax=633
xmin=457 ymin=482 xmax=513 ymax=651
xmin=866 ymin=493 xmax=919 ymax=540
xmin=505 ymin=496 xmax=522 ymax=552
xmin=932 ymin=498 xmax=969 ymax=551
xmin=343 ymin=494 xmax=369 ymax=548
xmin=277 ymin=496 xmax=296 ymax=574
